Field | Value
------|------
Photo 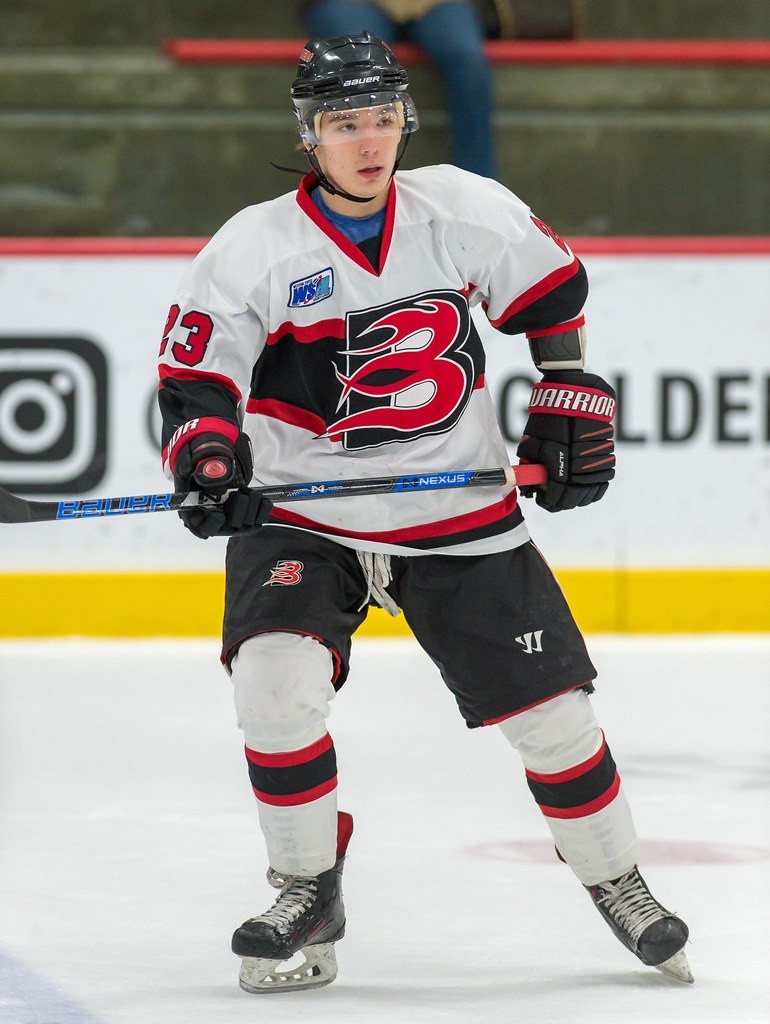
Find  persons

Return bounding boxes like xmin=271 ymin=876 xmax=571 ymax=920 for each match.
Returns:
xmin=296 ymin=0 xmax=494 ymax=179
xmin=156 ymin=31 xmax=701 ymax=995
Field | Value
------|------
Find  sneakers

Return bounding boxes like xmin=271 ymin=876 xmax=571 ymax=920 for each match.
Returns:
xmin=554 ymin=844 xmax=695 ymax=985
xmin=231 ymin=809 xmax=355 ymax=995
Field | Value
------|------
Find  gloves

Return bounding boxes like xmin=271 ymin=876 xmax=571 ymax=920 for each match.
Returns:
xmin=157 ymin=413 xmax=274 ymax=541
xmin=515 ymin=372 xmax=617 ymax=513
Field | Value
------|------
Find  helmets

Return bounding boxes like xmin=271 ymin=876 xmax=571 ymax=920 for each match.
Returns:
xmin=267 ymin=30 xmax=421 ymax=204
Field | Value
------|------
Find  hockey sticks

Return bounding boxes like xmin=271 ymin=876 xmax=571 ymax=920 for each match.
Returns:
xmin=1 ymin=463 xmax=549 ymax=526
